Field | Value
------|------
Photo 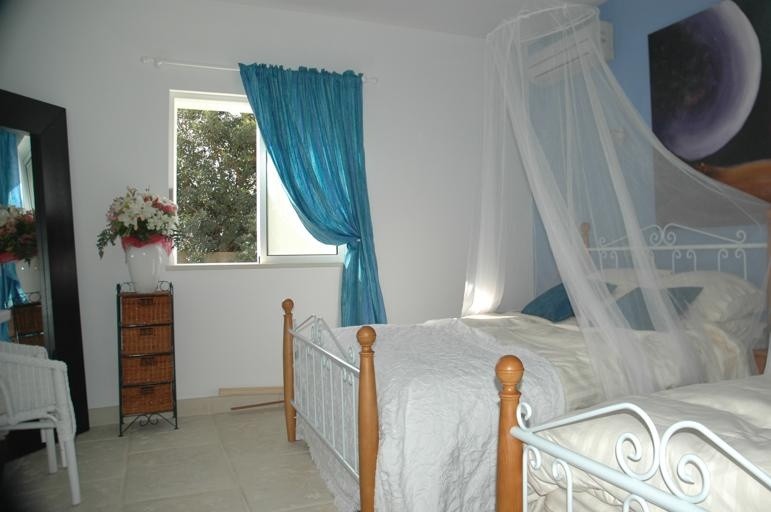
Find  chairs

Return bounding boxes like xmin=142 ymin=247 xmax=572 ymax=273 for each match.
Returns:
xmin=1 ymin=341 xmax=81 ymax=505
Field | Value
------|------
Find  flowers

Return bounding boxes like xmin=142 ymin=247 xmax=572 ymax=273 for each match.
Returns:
xmin=0 ymin=204 xmax=39 ymax=267
xmin=97 ymin=184 xmax=184 ymax=258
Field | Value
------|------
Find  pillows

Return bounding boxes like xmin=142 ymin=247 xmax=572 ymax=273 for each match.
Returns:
xmin=660 ymin=272 xmax=764 ymax=319
xmin=587 ymin=269 xmax=672 ymax=300
xmin=521 ymin=279 xmax=618 ymax=320
xmin=589 ymin=285 xmax=702 ymax=329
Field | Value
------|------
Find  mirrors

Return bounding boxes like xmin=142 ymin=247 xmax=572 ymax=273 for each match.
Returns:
xmin=1 ymin=88 xmax=89 ymax=462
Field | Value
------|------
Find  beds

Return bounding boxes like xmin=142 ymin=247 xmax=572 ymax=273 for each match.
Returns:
xmin=282 ymin=225 xmax=765 ymax=512
xmin=491 ymin=354 xmax=769 ymax=512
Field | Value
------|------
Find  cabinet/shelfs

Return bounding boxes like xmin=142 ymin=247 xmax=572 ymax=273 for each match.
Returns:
xmin=12 ymin=291 xmax=44 ymax=347
xmin=117 ymin=282 xmax=177 ymax=436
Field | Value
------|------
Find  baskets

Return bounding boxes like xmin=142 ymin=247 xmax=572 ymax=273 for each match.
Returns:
xmin=9 ymin=304 xmax=44 ymax=346
xmin=122 ymin=292 xmax=174 ymax=415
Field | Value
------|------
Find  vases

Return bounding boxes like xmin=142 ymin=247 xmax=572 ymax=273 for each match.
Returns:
xmin=121 ymin=236 xmax=171 ymax=294
xmin=15 ymin=256 xmax=43 ymax=303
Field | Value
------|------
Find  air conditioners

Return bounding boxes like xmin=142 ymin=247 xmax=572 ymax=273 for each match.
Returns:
xmin=526 ymin=20 xmax=614 ymax=87
